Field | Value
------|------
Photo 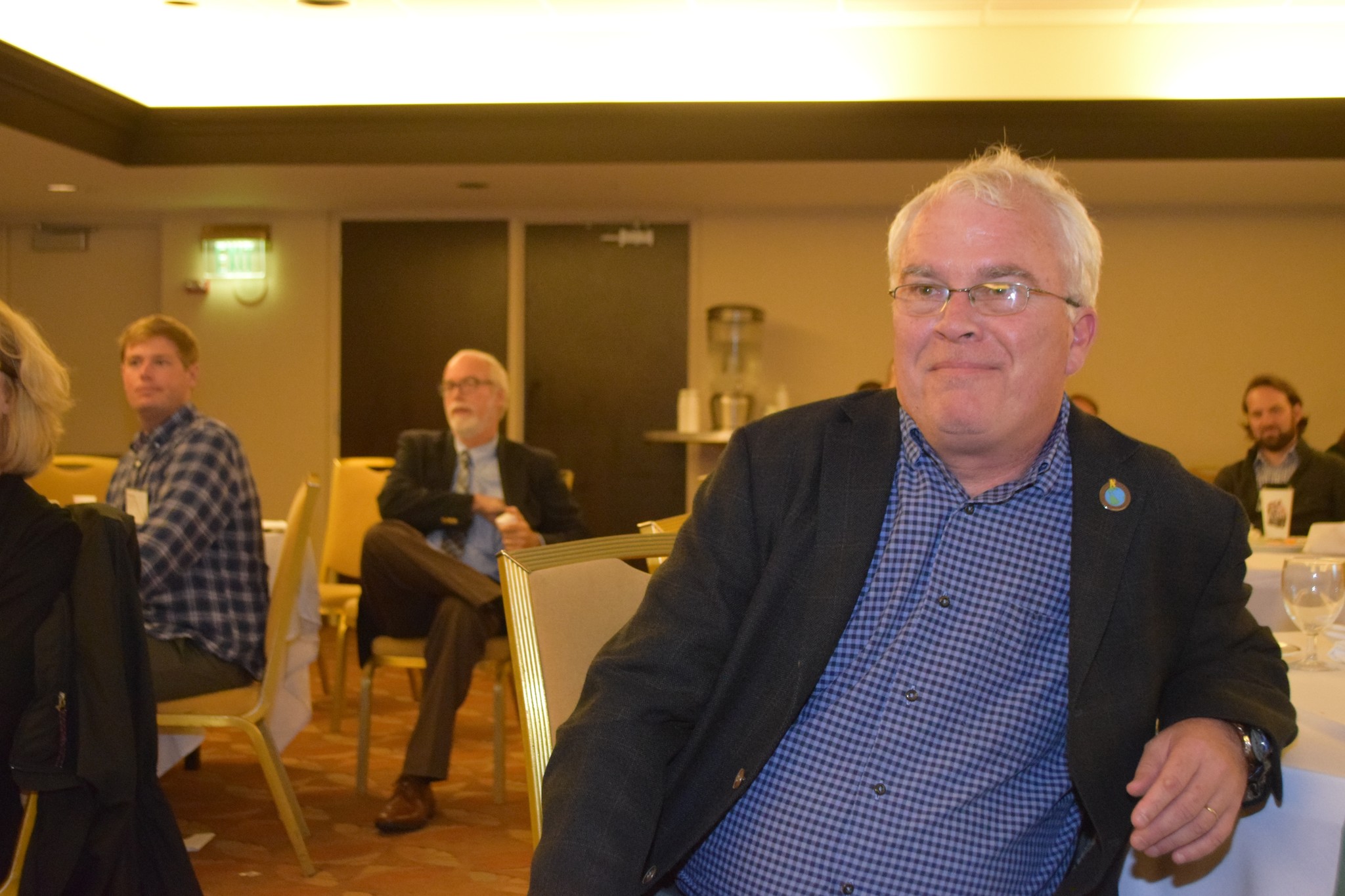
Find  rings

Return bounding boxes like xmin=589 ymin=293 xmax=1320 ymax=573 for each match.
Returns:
xmin=1204 ymin=805 xmax=1219 ymax=820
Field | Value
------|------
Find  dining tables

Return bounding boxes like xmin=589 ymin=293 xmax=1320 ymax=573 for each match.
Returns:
xmin=1113 ymin=536 xmax=1345 ymax=896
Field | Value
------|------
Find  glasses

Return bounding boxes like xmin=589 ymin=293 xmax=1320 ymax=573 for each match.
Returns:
xmin=888 ymin=283 xmax=1080 ymax=317
xmin=435 ymin=377 xmax=504 ymax=395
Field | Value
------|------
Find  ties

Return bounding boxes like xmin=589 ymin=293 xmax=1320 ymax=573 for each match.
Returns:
xmin=442 ymin=453 xmax=471 ymax=559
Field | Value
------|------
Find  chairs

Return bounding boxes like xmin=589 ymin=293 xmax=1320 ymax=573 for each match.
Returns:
xmin=29 ymin=456 xmax=696 ymax=880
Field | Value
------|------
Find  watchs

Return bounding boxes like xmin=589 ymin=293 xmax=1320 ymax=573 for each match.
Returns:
xmin=1229 ymin=721 xmax=1273 ymax=780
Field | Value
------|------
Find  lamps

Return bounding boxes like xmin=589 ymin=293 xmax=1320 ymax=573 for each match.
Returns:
xmin=209 ymin=226 xmax=269 ymax=305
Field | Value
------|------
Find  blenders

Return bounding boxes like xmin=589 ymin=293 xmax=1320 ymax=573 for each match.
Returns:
xmin=705 ymin=305 xmax=764 ymax=430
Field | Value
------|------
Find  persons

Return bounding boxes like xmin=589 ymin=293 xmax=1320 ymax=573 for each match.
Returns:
xmin=0 ymin=299 xmax=204 ymax=896
xmin=355 ymin=348 xmax=573 ymax=834
xmin=1212 ymin=374 xmax=1345 ymax=536
xmin=529 ymin=148 xmax=1298 ymax=896
xmin=105 ymin=314 xmax=271 ymax=704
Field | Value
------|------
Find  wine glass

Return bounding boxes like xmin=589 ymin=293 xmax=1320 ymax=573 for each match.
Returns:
xmin=1279 ymin=558 xmax=1344 ymax=672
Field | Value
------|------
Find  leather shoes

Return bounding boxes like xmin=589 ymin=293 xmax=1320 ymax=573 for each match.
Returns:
xmin=375 ymin=776 xmax=434 ymax=831
